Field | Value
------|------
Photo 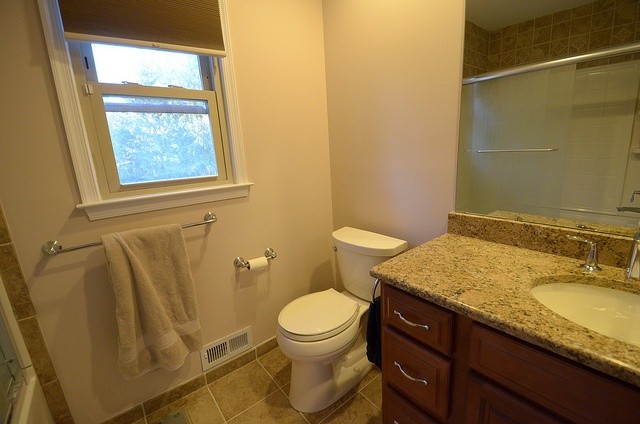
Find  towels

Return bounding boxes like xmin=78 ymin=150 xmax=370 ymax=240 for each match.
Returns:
xmin=100 ymin=224 xmax=203 ymax=380
xmin=365 ymin=296 xmax=382 ymax=367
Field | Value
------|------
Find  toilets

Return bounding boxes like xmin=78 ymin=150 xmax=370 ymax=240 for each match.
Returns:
xmin=274 ymin=225 xmax=409 ymax=416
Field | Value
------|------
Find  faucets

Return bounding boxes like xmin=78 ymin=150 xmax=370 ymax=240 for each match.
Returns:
xmin=624 ymin=232 xmax=639 ymax=279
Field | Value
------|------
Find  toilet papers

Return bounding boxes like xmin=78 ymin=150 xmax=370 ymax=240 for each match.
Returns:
xmin=246 ymin=253 xmax=268 ymax=273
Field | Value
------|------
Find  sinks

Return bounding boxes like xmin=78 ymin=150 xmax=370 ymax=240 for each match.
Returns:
xmin=530 ymin=272 xmax=640 ymax=349
